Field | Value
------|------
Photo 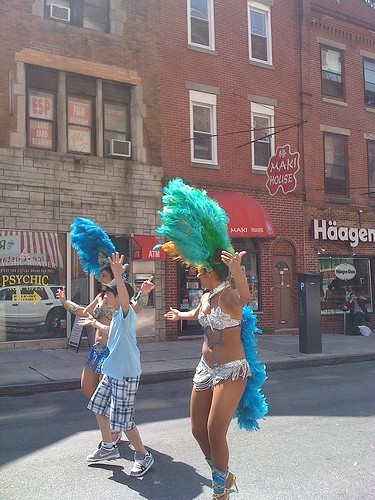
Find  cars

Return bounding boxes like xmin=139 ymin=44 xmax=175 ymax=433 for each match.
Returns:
xmin=0 ymin=285 xmax=67 ymax=335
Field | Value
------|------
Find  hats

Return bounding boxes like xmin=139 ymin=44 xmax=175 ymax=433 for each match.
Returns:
xmin=101 ymin=279 xmax=134 ymax=300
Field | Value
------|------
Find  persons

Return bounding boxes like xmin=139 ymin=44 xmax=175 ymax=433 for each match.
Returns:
xmin=151 ymin=177 xmax=269 ymax=500
xmin=340 ymin=292 xmax=372 ymax=335
xmin=78 ymin=251 xmax=155 ymax=477
xmin=55 ymin=217 xmax=155 ymax=449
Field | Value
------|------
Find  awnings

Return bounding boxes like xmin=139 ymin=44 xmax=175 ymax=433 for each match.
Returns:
xmin=209 ymin=191 xmax=276 ymax=239
xmin=0 ymin=230 xmax=63 ymax=268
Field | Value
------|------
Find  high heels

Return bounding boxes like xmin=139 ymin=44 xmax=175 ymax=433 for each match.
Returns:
xmin=210 ymin=471 xmax=239 ymax=500
xmin=111 ymin=429 xmax=122 ymax=445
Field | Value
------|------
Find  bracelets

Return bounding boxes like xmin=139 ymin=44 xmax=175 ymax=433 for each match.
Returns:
xmin=91 ymin=320 xmax=96 ymax=327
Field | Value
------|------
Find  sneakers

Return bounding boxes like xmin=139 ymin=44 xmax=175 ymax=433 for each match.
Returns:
xmin=130 ymin=449 xmax=154 ymax=477
xmin=86 ymin=440 xmax=120 ymax=462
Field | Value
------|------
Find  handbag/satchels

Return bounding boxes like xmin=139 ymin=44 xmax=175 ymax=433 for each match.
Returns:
xmin=358 ymin=325 xmax=372 ymax=336
